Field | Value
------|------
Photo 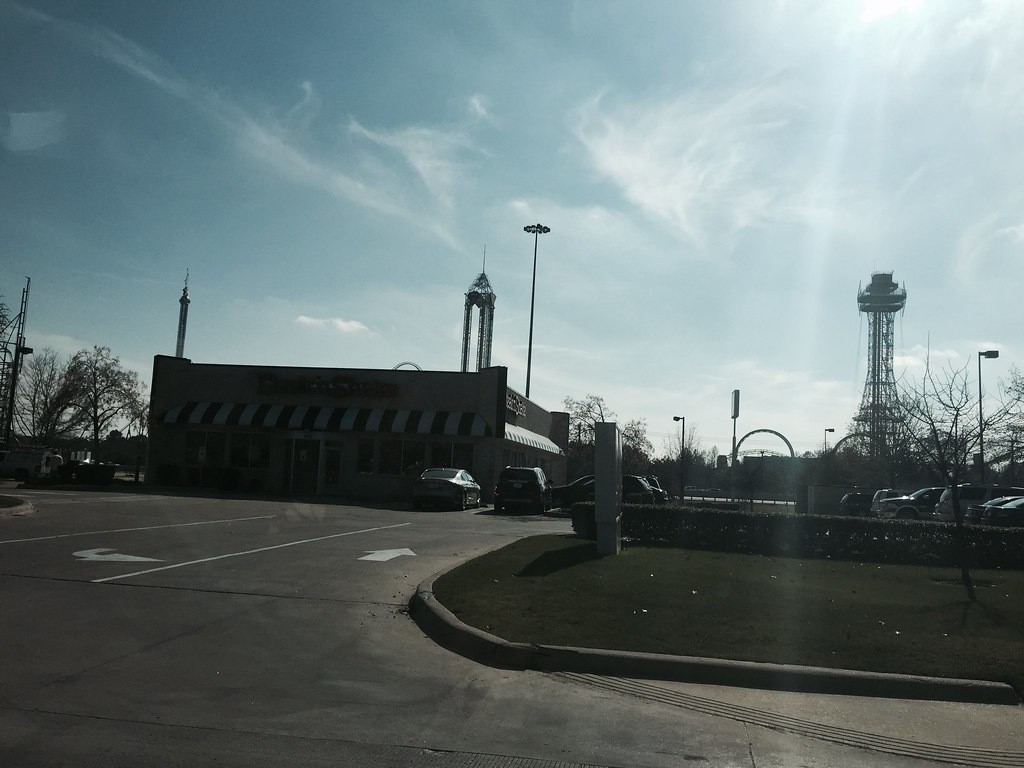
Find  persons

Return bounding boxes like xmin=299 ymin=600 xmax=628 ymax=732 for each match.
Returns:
xmin=548 ymin=475 xmax=554 ymax=486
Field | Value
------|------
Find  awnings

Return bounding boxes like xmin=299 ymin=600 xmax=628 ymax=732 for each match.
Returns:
xmin=504 ymin=422 xmax=566 ymax=456
xmin=158 ymin=400 xmax=493 ymax=437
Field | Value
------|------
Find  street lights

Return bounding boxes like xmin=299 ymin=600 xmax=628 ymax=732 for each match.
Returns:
xmin=4 ymin=346 xmax=33 ymax=453
xmin=825 ymin=429 xmax=835 ymax=453
xmin=673 ymin=416 xmax=685 ymax=505
xmin=525 ymin=224 xmax=551 ymax=398
xmin=978 ymin=350 xmax=999 ymax=483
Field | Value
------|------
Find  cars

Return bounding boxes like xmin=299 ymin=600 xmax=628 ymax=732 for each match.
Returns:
xmin=650 ymin=486 xmax=669 ymax=505
xmin=686 ymin=486 xmax=721 ymax=498
xmin=964 ymin=495 xmax=1024 ymax=527
xmin=413 ymin=467 xmax=482 ymax=511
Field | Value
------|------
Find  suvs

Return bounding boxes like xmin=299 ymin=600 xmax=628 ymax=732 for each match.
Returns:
xmin=492 ymin=465 xmax=554 ymax=514
xmin=932 ymin=484 xmax=1024 ymax=522
xmin=647 ymin=477 xmax=661 ymax=489
xmin=546 ymin=475 xmax=656 ymax=513
xmin=876 ymin=487 xmax=946 ymax=521
xmin=870 ymin=488 xmax=919 ymax=512
xmin=838 ymin=492 xmax=875 ymax=517
